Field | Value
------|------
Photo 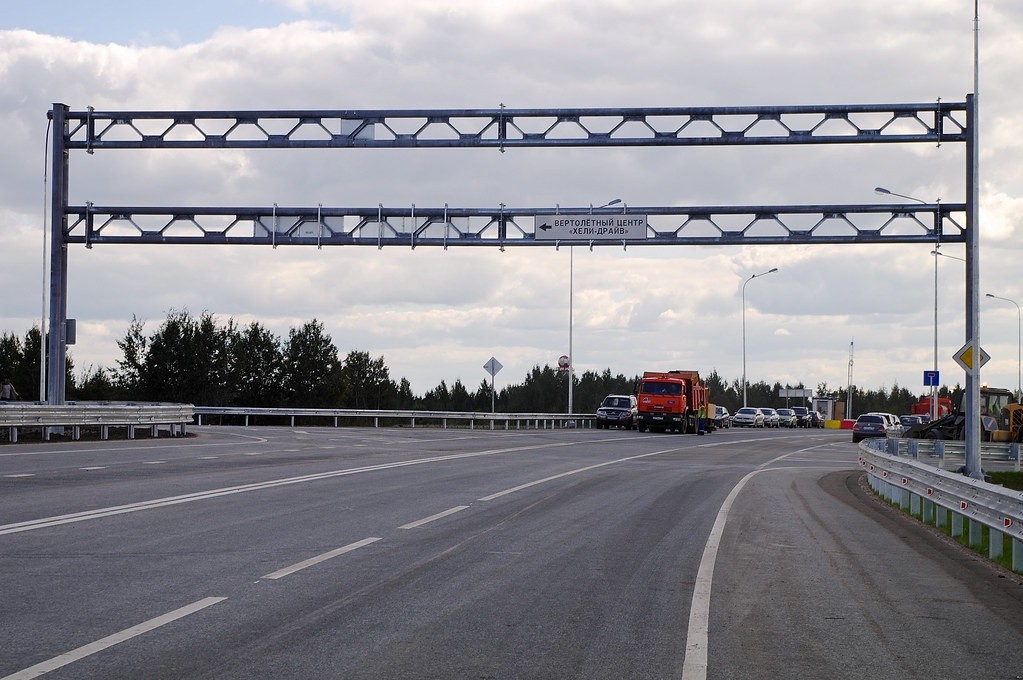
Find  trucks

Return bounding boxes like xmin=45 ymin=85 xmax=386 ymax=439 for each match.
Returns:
xmin=635 ymin=370 xmax=709 ymax=432
xmin=913 ymin=395 xmax=952 ymax=418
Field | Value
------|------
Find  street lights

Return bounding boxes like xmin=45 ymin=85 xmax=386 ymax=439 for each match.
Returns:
xmin=742 ymin=268 xmax=780 ymax=406
xmin=986 ymin=293 xmax=1020 ymax=404
xmin=878 ymin=187 xmax=938 ymax=422
xmin=569 ymin=197 xmax=622 ymax=415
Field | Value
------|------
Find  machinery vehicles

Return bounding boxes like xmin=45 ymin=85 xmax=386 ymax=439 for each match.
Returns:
xmin=932 ymin=387 xmax=1023 ymax=444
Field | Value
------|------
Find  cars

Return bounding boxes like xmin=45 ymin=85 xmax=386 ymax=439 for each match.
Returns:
xmin=776 ymin=408 xmax=798 ymax=429
xmin=901 ymin=414 xmax=931 ymax=433
xmin=852 ymin=415 xmax=891 ymax=443
xmin=712 ymin=406 xmax=731 ymax=428
xmin=809 ymin=410 xmax=824 ymax=428
xmin=733 ymin=407 xmax=765 ymax=428
xmin=870 ymin=412 xmax=904 ymax=434
xmin=759 ymin=408 xmax=781 ymax=428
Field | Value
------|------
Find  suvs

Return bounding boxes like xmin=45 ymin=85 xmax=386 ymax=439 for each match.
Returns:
xmin=595 ymin=394 xmax=639 ymax=430
xmin=791 ymin=407 xmax=812 ymax=429
